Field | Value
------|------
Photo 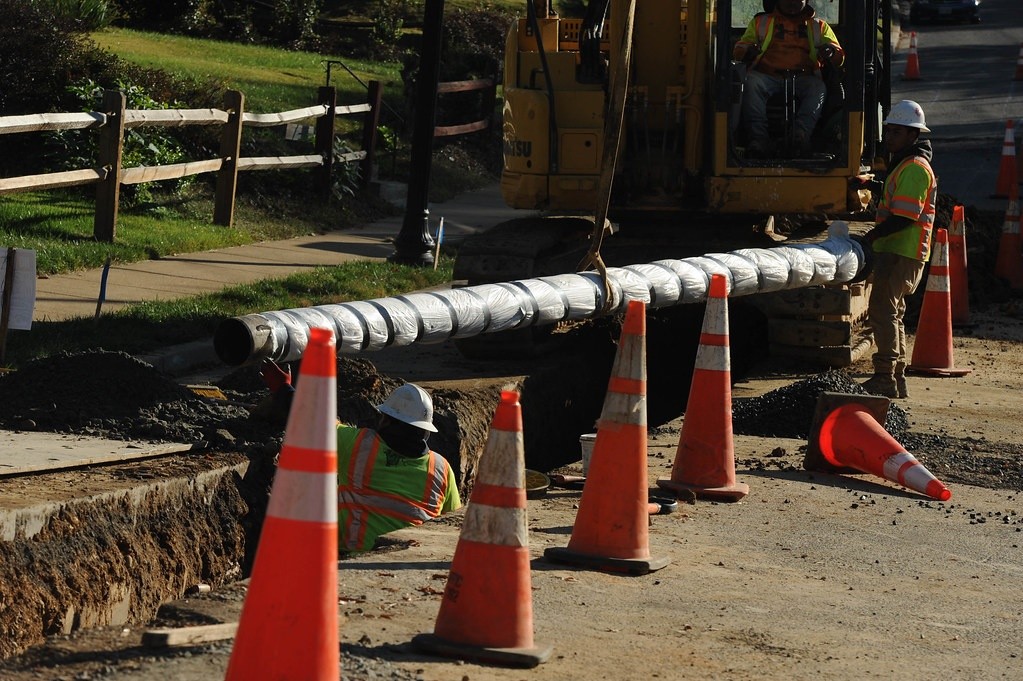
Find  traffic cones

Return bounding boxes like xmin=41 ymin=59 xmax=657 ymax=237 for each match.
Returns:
xmin=900 ymin=32 xmax=925 ymax=83
xmin=1010 ymin=38 xmax=1023 ymax=81
xmin=947 ymin=204 xmax=979 ymax=329
xmin=990 ymin=183 xmax=1023 ymax=291
xmin=226 ymin=327 xmax=341 ymax=681
xmin=540 ymin=300 xmax=667 ymax=576
xmin=905 ymin=228 xmax=972 ymax=377
xmin=802 ymin=389 xmax=952 ymax=500
xmin=659 ymin=272 xmax=751 ymax=499
xmin=411 ymin=390 xmax=550 ymax=666
xmin=988 ymin=120 xmax=1023 ymax=201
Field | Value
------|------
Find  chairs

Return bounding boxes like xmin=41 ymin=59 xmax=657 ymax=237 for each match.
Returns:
xmin=736 ymin=48 xmax=801 ymax=138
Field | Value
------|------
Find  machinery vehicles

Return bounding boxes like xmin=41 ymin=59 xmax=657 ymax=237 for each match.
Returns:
xmin=449 ymin=0 xmax=910 ymax=370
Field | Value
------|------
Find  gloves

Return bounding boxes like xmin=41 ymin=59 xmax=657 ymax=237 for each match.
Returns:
xmin=848 ymin=176 xmax=872 ymax=191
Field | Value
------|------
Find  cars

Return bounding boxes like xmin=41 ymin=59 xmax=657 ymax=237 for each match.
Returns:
xmin=907 ymin=0 xmax=983 ymax=26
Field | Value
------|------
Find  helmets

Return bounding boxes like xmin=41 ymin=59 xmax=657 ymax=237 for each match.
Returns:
xmin=883 ymin=99 xmax=931 ymax=133
xmin=374 ymin=382 xmax=437 ymax=433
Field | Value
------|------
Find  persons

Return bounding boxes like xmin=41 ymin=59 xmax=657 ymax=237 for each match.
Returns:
xmin=258 ymin=354 xmax=462 ymax=551
xmin=864 ymin=100 xmax=936 ymax=398
xmin=733 ymin=0 xmax=845 ymax=151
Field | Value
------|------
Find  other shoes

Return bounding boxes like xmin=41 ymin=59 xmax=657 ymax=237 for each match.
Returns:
xmin=859 ymin=371 xmax=908 ymax=399
xmin=791 ymin=130 xmax=810 ymax=152
xmin=746 ymin=138 xmax=770 ymax=163
